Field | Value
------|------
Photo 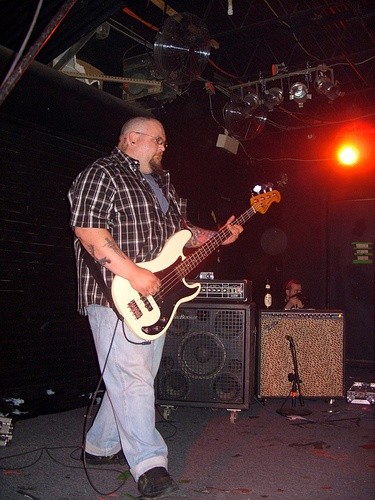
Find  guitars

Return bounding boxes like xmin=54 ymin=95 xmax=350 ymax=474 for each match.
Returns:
xmin=110 ymin=185 xmax=282 ymax=342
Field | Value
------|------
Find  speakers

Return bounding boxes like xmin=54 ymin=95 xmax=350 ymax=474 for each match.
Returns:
xmin=154 ymin=303 xmax=257 ymax=410
xmin=258 ymin=309 xmax=344 ymax=399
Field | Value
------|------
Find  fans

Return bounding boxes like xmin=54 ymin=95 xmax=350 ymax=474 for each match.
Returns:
xmin=153 ymin=12 xmax=210 ymax=85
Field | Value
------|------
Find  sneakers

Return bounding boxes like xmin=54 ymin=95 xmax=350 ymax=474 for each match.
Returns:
xmin=82 ymin=449 xmax=128 ymax=466
xmin=138 ymin=467 xmax=181 ymax=500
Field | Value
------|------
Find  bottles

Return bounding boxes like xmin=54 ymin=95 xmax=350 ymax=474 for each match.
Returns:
xmin=262 ymin=279 xmax=273 ymax=309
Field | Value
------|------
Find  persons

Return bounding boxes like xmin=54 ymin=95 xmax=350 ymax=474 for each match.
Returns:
xmin=280 ymin=278 xmax=304 ymax=310
xmin=68 ymin=116 xmax=244 ymax=500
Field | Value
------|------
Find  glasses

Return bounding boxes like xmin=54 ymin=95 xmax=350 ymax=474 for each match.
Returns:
xmin=136 ymin=132 xmax=169 ymax=150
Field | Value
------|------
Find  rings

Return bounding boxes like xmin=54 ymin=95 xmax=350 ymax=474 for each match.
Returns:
xmin=235 ymin=234 xmax=239 ymax=238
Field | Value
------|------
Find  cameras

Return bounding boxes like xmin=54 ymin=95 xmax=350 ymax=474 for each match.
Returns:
xmin=293 ymin=293 xmax=309 ymax=307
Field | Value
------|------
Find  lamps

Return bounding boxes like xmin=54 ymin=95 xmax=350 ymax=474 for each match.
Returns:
xmin=261 ymin=87 xmax=284 ymax=112
xmin=313 ymin=76 xmax=344 ymax=104
xmin=289 ymin=81 xmax=312 ymax=108
xmin=236 ymin=92 xmax=261 ymax=117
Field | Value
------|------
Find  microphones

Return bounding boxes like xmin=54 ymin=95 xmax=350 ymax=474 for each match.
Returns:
xmin=285 ymin=335 xmax=293 ymax=341
